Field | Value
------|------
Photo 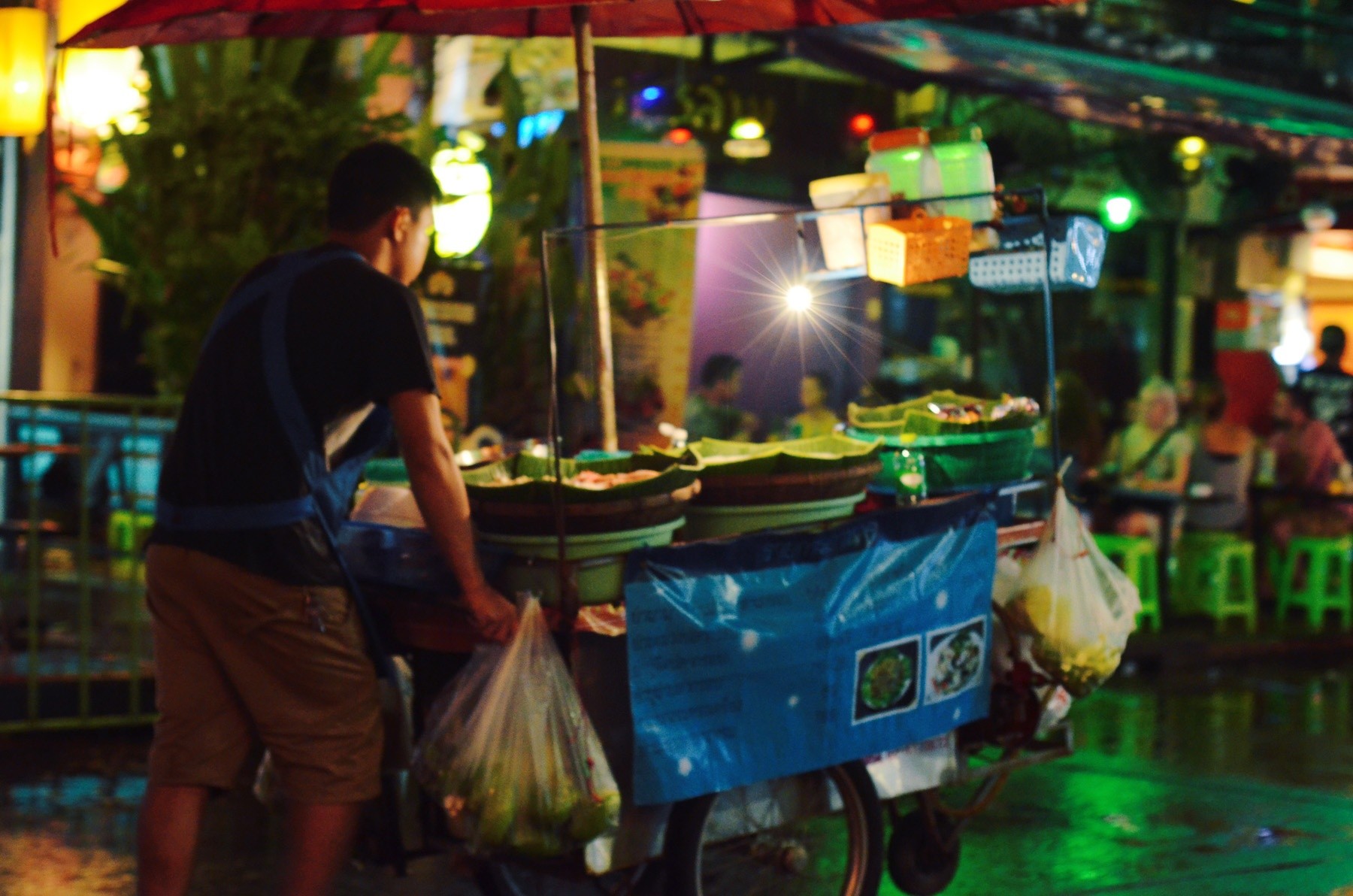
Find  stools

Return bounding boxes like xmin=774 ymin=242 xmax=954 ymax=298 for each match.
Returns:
xmin=1094 ymin=529 xmax=1164 ymax=632
xmin=1271 ymin=535 xmax=1353 ymax=633
xmin=1170 ymin=529 xmax=1259 ymax=636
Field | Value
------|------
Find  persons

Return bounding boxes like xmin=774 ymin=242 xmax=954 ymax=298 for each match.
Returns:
xmin=1036 ymin=325 xmax=1352 ymax=602
xmin=768 ymin=372 xmax=844 ymax=441
xmin=137 ymin=142 xmax=522 ymax=896
xmin=682 ymin=353 xmax=758 ymax=445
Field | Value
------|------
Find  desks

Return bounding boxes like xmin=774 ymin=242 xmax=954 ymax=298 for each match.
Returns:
xmin=1061 ymin=485 xmax=1238 ymax=634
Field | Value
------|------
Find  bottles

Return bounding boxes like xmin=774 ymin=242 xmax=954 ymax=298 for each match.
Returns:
xmin=864 ymin=123 xmax=998 ymax=222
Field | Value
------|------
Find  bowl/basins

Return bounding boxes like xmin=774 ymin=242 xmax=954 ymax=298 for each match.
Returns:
xmin=681 ymin=489 xmax=867 ymax=540
xmin=479 ymin=516 xmax=685 ymax=606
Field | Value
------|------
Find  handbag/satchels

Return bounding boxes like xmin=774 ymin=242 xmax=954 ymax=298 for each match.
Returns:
xmin=415 ymin=599 xmax=620 ymax=861
xmin=1003 ymin=475 xmax=1141 ymax=698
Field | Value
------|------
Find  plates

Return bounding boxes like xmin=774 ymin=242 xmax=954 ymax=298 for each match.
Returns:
xmin=861 ymin=652 xmax=911 ymax=707
xmin=927 ymin=632 xmax=984 ymax=701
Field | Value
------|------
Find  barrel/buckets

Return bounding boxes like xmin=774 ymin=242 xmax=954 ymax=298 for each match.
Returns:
xmin=809 ymin=172 xmax=891 ymax=270
